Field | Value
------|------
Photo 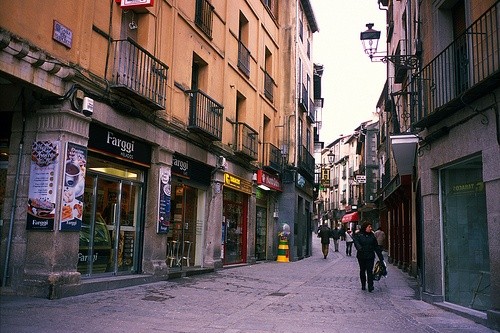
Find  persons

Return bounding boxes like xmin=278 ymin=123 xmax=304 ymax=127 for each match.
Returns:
xmin=353 ymin=221 xmax=384 ymax=293
xmin=371 ymin=225 xmax=386 ymax=253
xmin=317 ymin=222 xmax=360 ymax=259
xmin=101 ymin=195 xmax=126 ymax=245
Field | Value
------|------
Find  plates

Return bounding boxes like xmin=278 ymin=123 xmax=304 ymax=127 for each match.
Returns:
xmin=163 ymin=185 xmax=171 ymax=196
xmin=65 ymin=176 xmax=85 ymax=198
xmin=28 ymin=208 xmax=55 ymax=218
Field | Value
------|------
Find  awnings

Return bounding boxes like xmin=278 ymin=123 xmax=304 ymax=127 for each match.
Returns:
xmin=341 ymin=212 xmax=359 ymax=223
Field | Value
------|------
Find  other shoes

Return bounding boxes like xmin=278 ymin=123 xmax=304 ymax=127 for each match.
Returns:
xmin=362 ymin=286 xmax=365 ymax=290
xmin=324 ymin=255 xmax=326 ymax=259
xmin=349 ymin=253 xmax=351 ymax=256
xmin=346 ymin=253 xmax=348 ymax=256
xmin=369 ymin=287 xmax=374 ymax=292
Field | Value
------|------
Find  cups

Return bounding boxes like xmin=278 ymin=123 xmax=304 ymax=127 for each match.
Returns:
xmin=65 ymin=160 xmax=81 ymax=187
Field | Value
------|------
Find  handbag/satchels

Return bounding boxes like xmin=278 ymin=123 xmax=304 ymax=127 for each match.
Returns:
xmin=374 ymin=261 xmax=387 ymax=281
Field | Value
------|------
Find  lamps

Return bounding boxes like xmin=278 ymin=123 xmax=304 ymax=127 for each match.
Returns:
xmin=361 ymin=23 xmax=419 ymax=69
